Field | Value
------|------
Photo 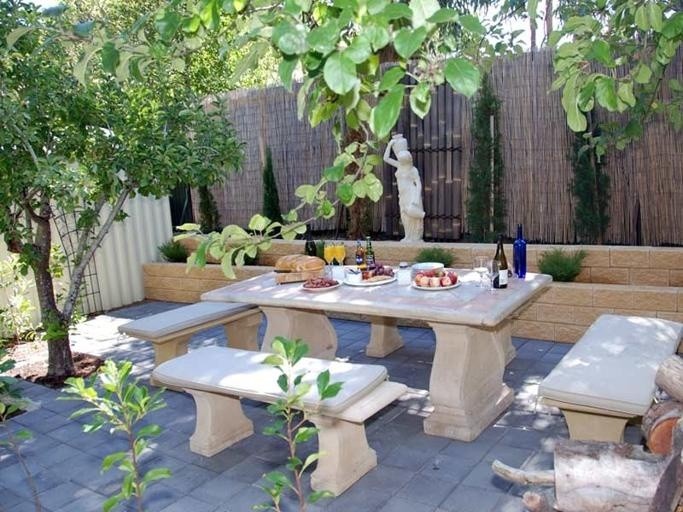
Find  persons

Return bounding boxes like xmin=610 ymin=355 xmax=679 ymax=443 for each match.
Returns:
xmin=382 ymin=138 xmax=425 ymax=246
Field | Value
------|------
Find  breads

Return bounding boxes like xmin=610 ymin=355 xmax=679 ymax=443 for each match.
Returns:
xmin=275 ymin=254 xmax=325 ymax=272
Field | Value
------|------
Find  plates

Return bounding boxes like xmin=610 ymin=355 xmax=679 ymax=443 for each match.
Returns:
xmin=410 ymin=260 xmax=443 ymax=273
xmin=342 ymin=273 xmax=398 ymax=287
xmin=410 ymin=280 xmax=461 ymax=291
xmin=300 ymin=279 xmax=342 ymax=293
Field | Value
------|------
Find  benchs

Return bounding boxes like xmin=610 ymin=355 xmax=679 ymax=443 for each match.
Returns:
xmin=115 ymin=299 xmax=264 ymax=391
xmin=534 ymin=308 xmax=683 ymax=449
xmin=151 ymin=343 xmax=408 ymax=501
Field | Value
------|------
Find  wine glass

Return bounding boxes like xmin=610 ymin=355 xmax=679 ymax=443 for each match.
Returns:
xmin=472 ymin=254 xmax=500 ymax=294
xmin=323 ymin=241 xmax=346 ymax=271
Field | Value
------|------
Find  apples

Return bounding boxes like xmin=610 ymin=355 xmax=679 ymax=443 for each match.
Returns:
xmin=415 ymin=270 xmax=458 ymax=287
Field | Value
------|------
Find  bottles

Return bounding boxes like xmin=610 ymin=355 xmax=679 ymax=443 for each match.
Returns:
xmin=304 ymin=222 xmax=316 ymax=256
xmin=364 ymin=235 xmax=375 ymax=265
xmin=491 ymin=233 xmax=509 ymax=289
xmin=354 ymin=238 xmax=364 ymax=266
xmin=513 ymin=222 xmax=527 ymax=280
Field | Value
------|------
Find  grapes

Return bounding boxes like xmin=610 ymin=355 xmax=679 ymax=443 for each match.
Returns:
xmin=374 ymin=261 xmax=394 ymax=277
